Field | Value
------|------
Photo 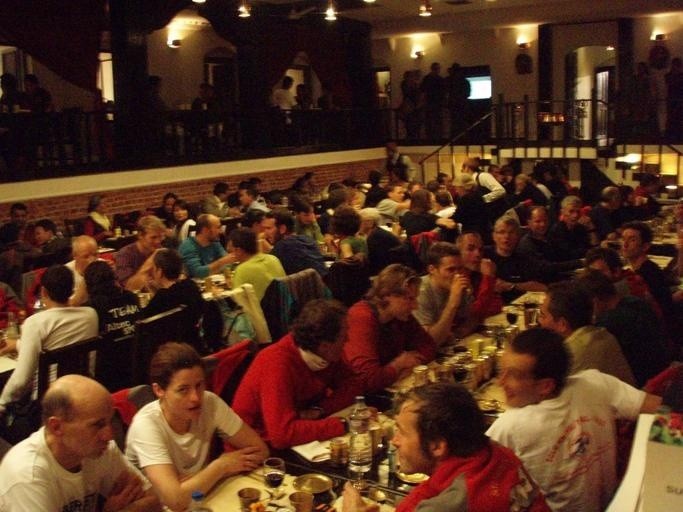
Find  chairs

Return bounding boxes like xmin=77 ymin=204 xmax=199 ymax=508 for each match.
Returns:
xmin=0 ymin=187 xmax=683 ymax=442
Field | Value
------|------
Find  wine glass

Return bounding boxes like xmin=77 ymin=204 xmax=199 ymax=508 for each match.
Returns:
xmin=261 ymin=456 xmax=284 ymax=505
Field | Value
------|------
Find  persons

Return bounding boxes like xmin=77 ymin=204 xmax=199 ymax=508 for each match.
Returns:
xmin=0 ymin=157 xmax=683 ymax=512
xmin=396 ymin=60 xmax=470 ymax=145
xmin=622 ymin=61 xmax=658 ymax=137
xmin=0 ymin=73 xmax=23 ymax=164
xmin=660 ymin=58 xmax=683 ymax=142
xmin=271 ymin=76 xmax=351 ymax=146
xmin=382 ymin=141 xmax=415 ymax=183
xmin=18 ymin=74 xmax=62 ymax=164
xmin=145 ymin=74 xmax=181 ymax=156
xmin=192 ymin=84 xmax=219 ymax=143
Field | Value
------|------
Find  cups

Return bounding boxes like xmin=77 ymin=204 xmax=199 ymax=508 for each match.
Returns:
xmin=453 ymin=338 xmax=468 ymax=353
xmin=289 ymin=491 xmax=315 ymax=512
xmin=453 ymin=363 xmax=469 ymax=384
xmin=506 ymin=306 xmax=519 ymax=325
xmin=124 ymin=228 xmax=129 ymax=237
xmin=524 ymin=295 xmax=540 ymax=309
xmin=280 ymin=196 xmax=288 ymax=206
xmin=237 ymin=488 xmax=262 ymax=512
xmin=527 ymin=310 xmax=540 ymax=328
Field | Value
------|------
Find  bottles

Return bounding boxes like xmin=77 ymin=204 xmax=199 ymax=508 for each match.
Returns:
xmin=6 ymin=312 xmax=18 ymax=336
xmin=188 ymin=490 xmax=208 ymax=511
xmin=114 ymin=225 xmax=121 ymax=238
xmin=348 ymin=396 xmax=374 ymax=474
xmin=463 ymin=286 xmax=477 ymax=306
xmin=224 ymin=266 xmax=231 ymax=287
xmin=18 ymin=310 xmax=26 ymax=324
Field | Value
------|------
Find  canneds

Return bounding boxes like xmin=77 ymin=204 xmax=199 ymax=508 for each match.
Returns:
xmin=437 ymin=353 xmax=491 ymax=391
xmin=412 ymin=364 xmax=430 ymax=391
xmin=331 ymin=437 xmax=347 ymax=465
xmin=455 ymin=272 xmax=473 ymax=294
xmin=506 ymin=324 xmax=519 ymax=345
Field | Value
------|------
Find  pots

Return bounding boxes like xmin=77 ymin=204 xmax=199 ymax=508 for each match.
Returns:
xmin=283 ymin=473 xmax=343 ymax=503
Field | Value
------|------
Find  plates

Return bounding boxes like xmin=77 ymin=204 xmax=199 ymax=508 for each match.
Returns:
xmin=397 ymin=468 xmax=429 ymax=485
xmin=473 ymin=399 xmax=500 ymax=414
